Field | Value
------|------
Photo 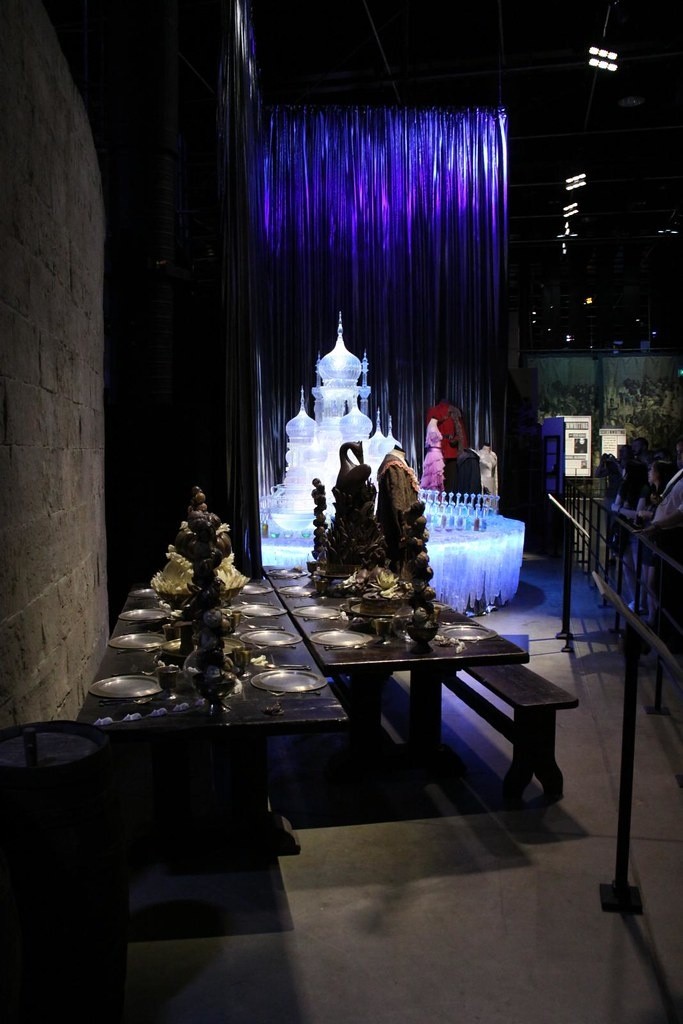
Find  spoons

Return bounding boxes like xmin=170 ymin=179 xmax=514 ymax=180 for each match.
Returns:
xmin=304 ymin=616 xmax=340 ymax=620
xmin=325 ymin=644 xmax=366 ymax=649
xmin=248 ymin=624 xmax=283 ymax=629
xmin=99 ymin=698 xmax=152 ymax=704
xmin=117 ymin=647 xmax=159 ymax=654
xmin=266 ymin=663 xmax=309 ymax=668
xmin=442 ymin=621 xmax=477 ymax=625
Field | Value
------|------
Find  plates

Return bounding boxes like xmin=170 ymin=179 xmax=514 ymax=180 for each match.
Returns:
xmin=437 ymin=625 xmax=497 ymax=640
xmin=118 ymin=609 xmax=170 ymax=620
xmin=310 ymin=631 xmax=372 ymax=647
xmin=89 ymin=675 xmax=163 ymax=697
xmin=236 ymin=604 xmax=288 ymax=616
xmin=278 ymin=587 xmax=317 ymax=595
xmin=251 ymin=670 xmax=328 ymax=692
xmin=240 ymin=631 xmax=302 ymax=645
xmin=129 ymin=589 xmax=159 ymax=597
xmin=292 ymin=606 xmax=340 ymax=617
xmin=109 ymin=633 xmax=166 ymax=648
xmin=241 ymin=585 xmax=274 ymax=594
xmin=266 ymin=569 xmax=307 ymax=576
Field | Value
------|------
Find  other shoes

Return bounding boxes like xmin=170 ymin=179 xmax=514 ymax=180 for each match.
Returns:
xmin=627 ymin=601 xmax=635 ymax=613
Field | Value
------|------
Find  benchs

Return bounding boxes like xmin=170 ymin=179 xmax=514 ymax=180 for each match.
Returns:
xmin=442 ymin=660 xmax=579 ymax=810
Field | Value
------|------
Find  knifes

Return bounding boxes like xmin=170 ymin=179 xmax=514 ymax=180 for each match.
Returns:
xmin=245 ymin=628 xmax=284 ymax=631
xmin=311 ymin=628 xmax=350 ymax=633
xmin=263 ymin=668 xmax=311 ymax=671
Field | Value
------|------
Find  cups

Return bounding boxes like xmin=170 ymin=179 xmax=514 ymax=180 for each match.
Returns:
xmin=162 ymin=624 xmax=179 ymax=640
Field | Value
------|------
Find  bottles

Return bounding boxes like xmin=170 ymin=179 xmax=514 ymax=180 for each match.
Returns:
xmin=418 ymin=490 xmax=500 ymax=532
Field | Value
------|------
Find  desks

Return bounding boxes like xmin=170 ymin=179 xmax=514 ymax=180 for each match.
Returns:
xmin=70 ymin=563 xmax=530 ymax=898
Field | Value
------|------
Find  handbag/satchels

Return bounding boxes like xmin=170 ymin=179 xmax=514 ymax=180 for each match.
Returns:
xmin=605 ymin=514 xmax=628 ymax=557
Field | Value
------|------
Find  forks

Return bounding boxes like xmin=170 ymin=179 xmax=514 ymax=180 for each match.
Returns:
xmin=253 ymin=642 xmax=296 ymax=649
xmin=241 ymin=613 xmax=279 ymax=619
xmin=123 ymin=630 xmax=160 ymax=634
xmin=467 ymin=639 xmax=503 ymax=643
xmin=267 ymin=690 xmax=321 ymax=696
xmin=111 ymin=671 xmax=157 ymax=676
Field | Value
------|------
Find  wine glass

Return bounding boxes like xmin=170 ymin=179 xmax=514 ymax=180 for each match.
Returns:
xmin=406 ymin=622 xmax=439 ymax=654
xmin=191 ymin=673 xmax=235 ymax=715
xmin=158 ymin=667 xmax=179 ymax=700
xmin=306 ymin=561 xmax=318 ymax=578
xmin=348 ymin=597 xmax=364 ymax=623
xmin=227 ymin=611 xmax=241 ymax=635
xmin=374 ymin=618 xmax=392 ymax=644
xmin=315 ymin=581 xmax=327 ymax=597
xmin=231 ymin=646 xmax=252 ymax=677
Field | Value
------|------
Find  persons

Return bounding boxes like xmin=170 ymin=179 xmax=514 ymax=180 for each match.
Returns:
xmin=426 ymin=399 xmax=466 ymax=502
xmin=477 ymin=444 xmax=498 ymax=508
xmin=594 ymin=438 xmax=683 ymax=658
xmin=419 ymin=416 xmax=445 ymax=502
